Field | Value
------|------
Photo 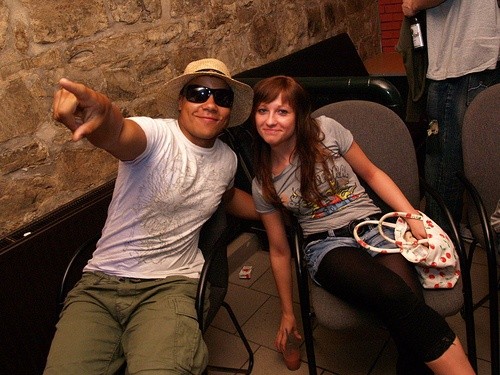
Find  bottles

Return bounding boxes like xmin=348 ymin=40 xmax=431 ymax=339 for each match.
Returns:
xmin=408 ymin=12 xmax=427 ymax=53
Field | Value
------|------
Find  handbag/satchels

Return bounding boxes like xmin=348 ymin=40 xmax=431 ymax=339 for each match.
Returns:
xmin=353 ymin=209 xmax=460 ymax=289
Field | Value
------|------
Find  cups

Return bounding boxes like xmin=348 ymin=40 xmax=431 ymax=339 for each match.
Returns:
xmin=281 ymin=335 xmax=302 ymax=370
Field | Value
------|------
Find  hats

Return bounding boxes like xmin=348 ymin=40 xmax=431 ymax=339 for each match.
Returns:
xmin=159 ymin=58 xmax=253 ymax=129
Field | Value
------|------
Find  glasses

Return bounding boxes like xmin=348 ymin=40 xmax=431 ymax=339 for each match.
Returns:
xmin=180 ymin=84 xmax=234 ymax=110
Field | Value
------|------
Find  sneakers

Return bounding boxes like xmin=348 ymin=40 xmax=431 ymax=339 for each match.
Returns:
xmin=459 ymin=222 xmax=484 ymax=248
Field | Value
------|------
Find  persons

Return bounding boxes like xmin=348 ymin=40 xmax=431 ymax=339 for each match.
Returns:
xmin=251 ymin=74 xmax=477 ymax=375
xmin=400 ymin=0 xmax=500 ymax=260
xmin=42 ymin=57 xmax=292 ymax=375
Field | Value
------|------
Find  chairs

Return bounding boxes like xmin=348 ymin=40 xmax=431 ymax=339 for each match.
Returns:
xmin=458 ymin=82 xmax=499 ymax=375
xmin=291 ymin=101 xmax=478 ymax=375
xmin=58 ymin=211 xmax=254 ymax=374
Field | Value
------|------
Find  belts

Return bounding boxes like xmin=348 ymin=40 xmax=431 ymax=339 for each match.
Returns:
xmin=304 ymin=213 xmax=387 ymax=249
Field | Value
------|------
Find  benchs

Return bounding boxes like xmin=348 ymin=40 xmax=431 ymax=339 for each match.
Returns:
xmin=361 ymin=2 xmax=407 ymax=77
